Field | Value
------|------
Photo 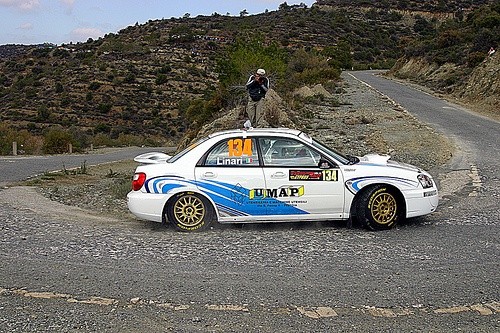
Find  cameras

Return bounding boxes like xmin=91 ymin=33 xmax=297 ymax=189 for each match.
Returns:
xmin=259 ymin=76 xmax=265 ymax=82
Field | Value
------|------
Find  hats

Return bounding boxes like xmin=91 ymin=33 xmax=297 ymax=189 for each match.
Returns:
xmin=257 ymin=69 xmax=265 ymax=75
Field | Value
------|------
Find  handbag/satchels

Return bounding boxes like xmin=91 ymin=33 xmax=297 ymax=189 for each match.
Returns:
xmin=250 ymin=95 xmax=261 ymax=101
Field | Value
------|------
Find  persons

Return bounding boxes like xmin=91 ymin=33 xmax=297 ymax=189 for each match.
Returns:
xmin=246 ymin=68 xmax=269 ymax=128
xmin=488 ymin=46 xmax=496 ymax=57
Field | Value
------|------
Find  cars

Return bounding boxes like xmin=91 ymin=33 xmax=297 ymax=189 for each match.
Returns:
xmin=127 ymin=128 xmax=438 ymax=233
